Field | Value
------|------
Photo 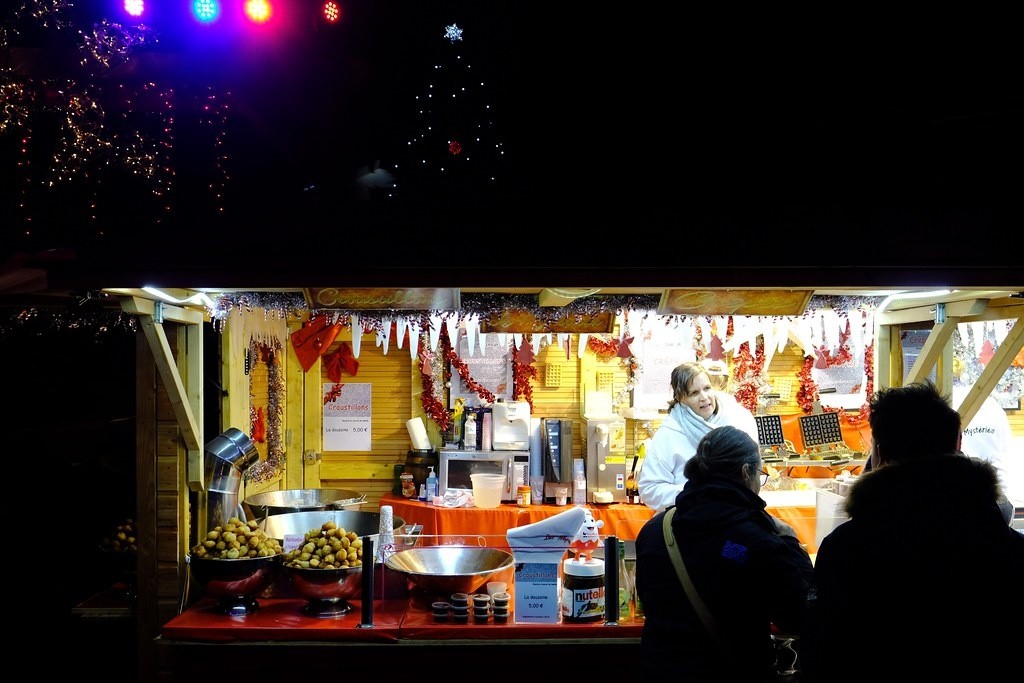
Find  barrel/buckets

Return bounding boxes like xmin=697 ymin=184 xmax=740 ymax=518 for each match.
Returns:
xmin=405 ymin=450 xmax=439 ymax=498
xmin=469 ymin=473 xmax=506 ymax=509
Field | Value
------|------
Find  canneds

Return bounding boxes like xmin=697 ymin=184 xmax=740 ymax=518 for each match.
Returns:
xmin=432 ymin=593 xmax=510 ymax=624
xmin=561 ymin=556 xmax=606 ymax=623
xmin=517 ymin=486 xmax=531 ymax=507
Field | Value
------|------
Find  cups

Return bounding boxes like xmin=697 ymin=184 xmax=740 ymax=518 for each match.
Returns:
xmin=481 ymin=412 xmax=492 ymax=451
xmin=530 ymin=475 xmax=545 ymax=506
xmin=554 ymin=487 xmax=568 ymax=506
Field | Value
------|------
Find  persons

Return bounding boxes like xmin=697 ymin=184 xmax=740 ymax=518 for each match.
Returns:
xmin=636 ymin=426 xmax=811 ymax=682
xmin=812 ymin=377 xmax=1023 ymax=683
xmin=639 ymin=364 xmax=759 ymax=509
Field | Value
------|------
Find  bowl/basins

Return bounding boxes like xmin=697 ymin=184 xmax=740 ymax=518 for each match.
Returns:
xmin=384 ymin=544 xmax=515 ymax=595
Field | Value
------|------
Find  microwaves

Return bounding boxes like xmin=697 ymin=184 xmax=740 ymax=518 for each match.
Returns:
xmin=439 ymin=450 xmax=530 ymax=502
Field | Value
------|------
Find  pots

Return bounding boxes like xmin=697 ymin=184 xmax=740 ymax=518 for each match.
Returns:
xmin=186 ymin=550 xmax=286 ymax=616
xmin=277 ymin=558 xmax=378 ymax=617
xmin=244 ymin=490 xmax=406 ymax=559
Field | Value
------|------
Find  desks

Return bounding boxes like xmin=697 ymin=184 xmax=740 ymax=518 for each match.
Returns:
xmin=378 ymin=491 xmax=819 ymax=566
xmin=151 ymin=596 xmax=644 ymax=651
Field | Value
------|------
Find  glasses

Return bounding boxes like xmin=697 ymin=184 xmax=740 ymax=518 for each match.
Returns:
xmin=755 ymin=469 xmax=770 ymax=486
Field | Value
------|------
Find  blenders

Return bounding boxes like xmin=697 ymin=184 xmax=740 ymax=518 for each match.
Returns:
xmin=580 ymin=415 xmax=629 ymax=506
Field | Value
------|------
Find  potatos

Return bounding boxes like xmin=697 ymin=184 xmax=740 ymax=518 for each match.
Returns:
xmin=189 ymin=516 xmax=282 ymax=558
xmin=281 ymin=521 xmax=363 ymax=569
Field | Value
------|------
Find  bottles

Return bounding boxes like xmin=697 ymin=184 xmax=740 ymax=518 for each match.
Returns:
xmin=619 ymin=539 xmax=631 ymax=620
xmin=393 ymin=462 xmax=438 ymax=502
xmin=574 ymin=471 xmax=587 ymax=506
xmin=635 ymin=591 xmax=645 ymax=622
xmin=628 ymin=490 xmax=639 ymax=505
xmin=464 ymin=415 xmax=477 ymax=450
